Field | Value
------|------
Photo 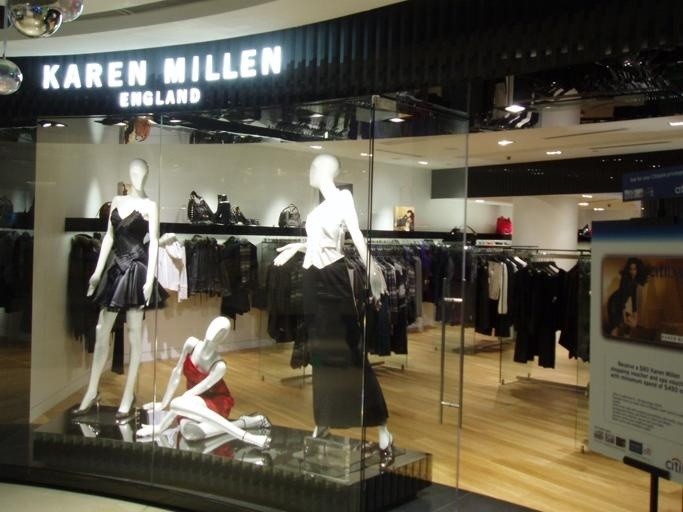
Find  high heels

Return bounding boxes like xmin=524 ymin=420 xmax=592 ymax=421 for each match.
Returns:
xmin=237 ymin=411 xmax=272 ymax=430
xmin=69 ymin=391 xmax=101 ymax=416
xmin=114 ymin=395 xmax=135 ymax=419
xmin=240 ymin=431 xmax=271 ymax=451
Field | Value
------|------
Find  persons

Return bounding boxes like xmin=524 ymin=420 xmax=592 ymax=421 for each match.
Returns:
xmin=273 ymin=153 xmax=397 ymax=473
xmin=134 ymin=313 xmax=272 ymax=454
xmin=606 ymin=257 xmax=648 ymax=339
xmin=72 ymin=159 xmax=157 ymax=420
xmin=396 ymin=210 xmax=414 ymax=232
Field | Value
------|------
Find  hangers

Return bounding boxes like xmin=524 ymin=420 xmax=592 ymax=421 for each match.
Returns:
xmin=475 ymin=248 xmax=585 ymax=277
xmin=374 ymin=245 xmax=401 ymax=267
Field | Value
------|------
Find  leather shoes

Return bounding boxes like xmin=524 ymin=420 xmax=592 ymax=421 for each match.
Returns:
xmin=305 ymin=426 xmax=331 ymax=456
xmin=378 ymin=431 xmax=395 ymax=471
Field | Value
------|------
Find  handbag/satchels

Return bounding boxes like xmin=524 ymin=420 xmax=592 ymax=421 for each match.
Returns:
xmin=187 ymin=191 xmax=213 ymax=221
xmin=214 ymin=195 xmax=232 ymax=224
xmin=496 ymin=216 xmax=511 ymax=236
xmin=232 ymin=205 xmax=249 ymax=225
xmin=278 ymin=203 xmax=300 ymax=228
